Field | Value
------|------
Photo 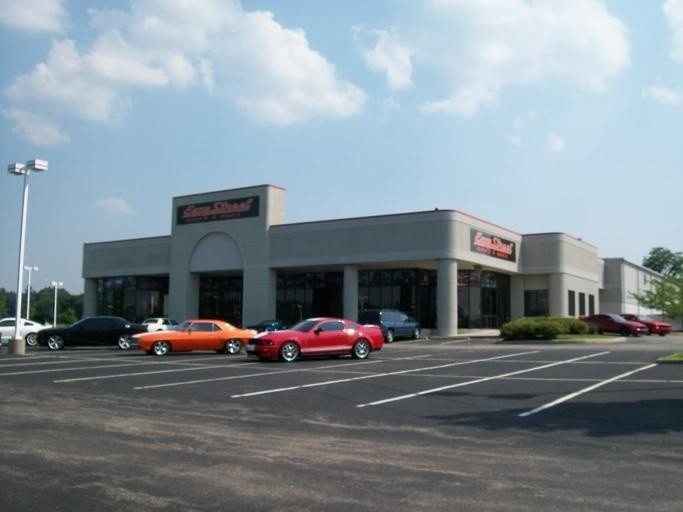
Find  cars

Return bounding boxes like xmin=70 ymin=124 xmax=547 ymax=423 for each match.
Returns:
xmin=245 ymin=317 xmax=386 ymax=361
xmin=131 ymin=318 xmax=258 ymax=356
xmin=618 ymin=312 xmax=673 ymax=337
xmin=35 ymin=315 xmax=149 ymax=351
xmin=578 ymin=312 xmax=651 ymax=339
xmin=1 ymin=317 xmax=53 ymax=346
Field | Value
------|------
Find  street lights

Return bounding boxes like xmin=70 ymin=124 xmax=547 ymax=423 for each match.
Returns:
xmin=49 ymin=281 xmax=64 ymax=328
xmin=22 ymin=265 xmax=39 ymax=321
xmin=6 ymin=158 xmax=50 ymax=359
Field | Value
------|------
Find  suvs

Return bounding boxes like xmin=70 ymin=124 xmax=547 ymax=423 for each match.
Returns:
xmin=358 ymin=309 xmax=422 ymax=344
xmin=141 ymin=318 xmax=180 ymax=332
